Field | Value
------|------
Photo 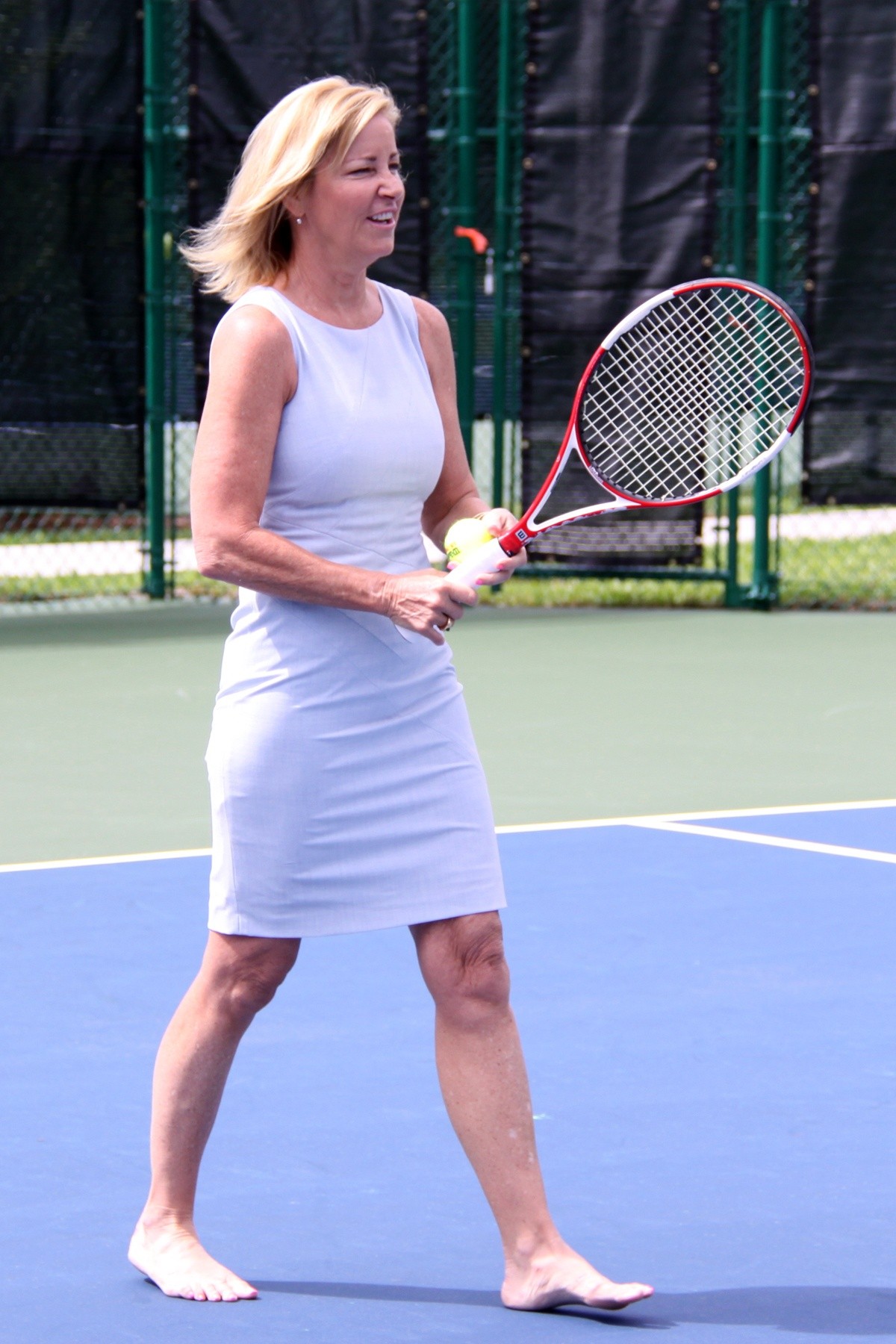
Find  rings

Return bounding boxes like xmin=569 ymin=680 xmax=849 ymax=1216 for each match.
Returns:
xmin=438 ymin=617 xmax=452 ymax=631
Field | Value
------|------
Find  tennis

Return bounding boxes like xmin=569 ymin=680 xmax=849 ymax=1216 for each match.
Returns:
xmin=443 ymin=519 xmax=492 ymax=565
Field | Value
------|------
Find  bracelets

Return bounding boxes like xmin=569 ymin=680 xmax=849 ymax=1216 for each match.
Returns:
xmin=473 ymin=512 xmax=485 ymax=519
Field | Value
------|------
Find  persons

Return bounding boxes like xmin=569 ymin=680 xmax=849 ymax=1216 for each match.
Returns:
xmin=128 ymin=77 xmax=651 ymax=1312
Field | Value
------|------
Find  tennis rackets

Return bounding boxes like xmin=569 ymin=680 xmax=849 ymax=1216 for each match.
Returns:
xmin=419 ymin=276 xmax=817 ymax=631
